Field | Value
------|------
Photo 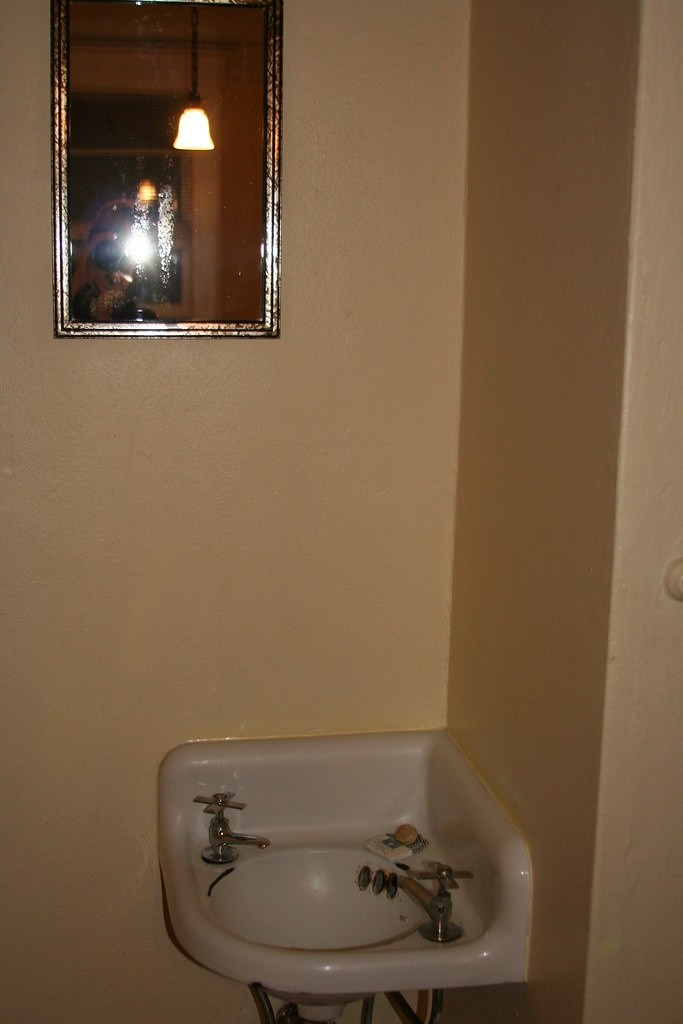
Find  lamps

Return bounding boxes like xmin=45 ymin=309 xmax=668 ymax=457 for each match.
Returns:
xmin=173 ymin=6 xmax=215 ymax=151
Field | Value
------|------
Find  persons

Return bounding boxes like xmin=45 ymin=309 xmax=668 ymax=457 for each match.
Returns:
xmin=70 ymin=178 xmax=174 ymax=323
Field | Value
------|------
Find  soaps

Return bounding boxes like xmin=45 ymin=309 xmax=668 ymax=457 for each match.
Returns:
xmin=394 ymin=824 xmax=418 ymax=846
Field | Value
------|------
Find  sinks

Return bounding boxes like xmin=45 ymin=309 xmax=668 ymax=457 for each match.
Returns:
xmin=205 ymin=846 xmax=431 ymax=952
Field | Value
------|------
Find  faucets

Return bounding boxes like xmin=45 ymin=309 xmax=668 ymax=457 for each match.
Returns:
xmin=192 ymin=791 xmax=271 ymax=865
xmin=397 ymin=861 xmax=465 ymax=943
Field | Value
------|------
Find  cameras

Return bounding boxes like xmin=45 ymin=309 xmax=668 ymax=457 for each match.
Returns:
xmin=94 ymin=238 xmax=128 ymax=271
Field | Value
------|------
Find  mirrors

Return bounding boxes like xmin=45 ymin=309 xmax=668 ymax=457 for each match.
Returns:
xmin=51 ymin=0 xmax=284 ymax=340
